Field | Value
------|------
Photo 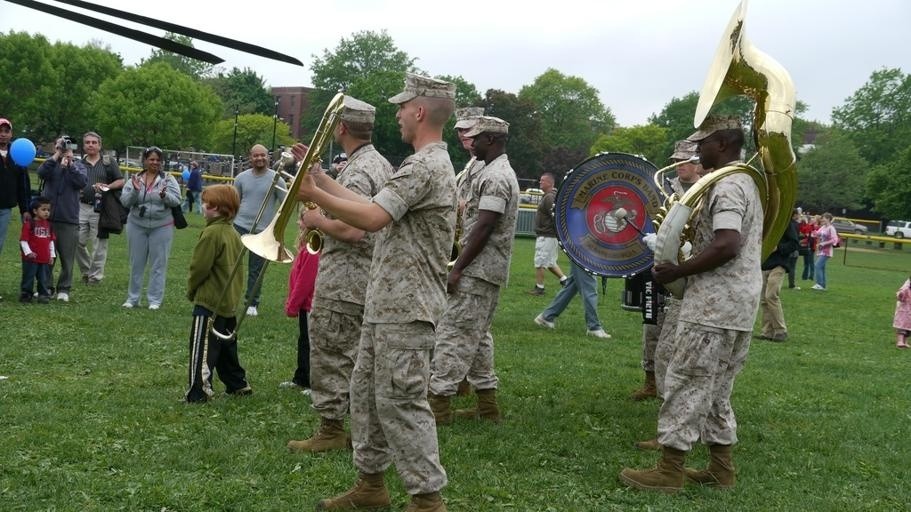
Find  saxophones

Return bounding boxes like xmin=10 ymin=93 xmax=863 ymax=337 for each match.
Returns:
xmin=297 ymin=156 xmax=325 ymax=254
xmin=447 ymin=188 xmax=465 ymax=267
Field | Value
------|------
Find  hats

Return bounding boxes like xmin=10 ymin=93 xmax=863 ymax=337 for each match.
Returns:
xmin=0 ymin=117 xmax=13 ymax=130
xmin=386 ymin=71 xmax=459 ymax=105
xmin=685 ymin=106 xmax=743 ymax=142
xmin=338 ymin=95 xmax=377 ymax=123
xmin=452 ymin=106 xmax=486 ymax=131
xmin=669 ymin=139 xmax=699 ymax=162
xmin=462 ymin=114 xmax=512 ymax=138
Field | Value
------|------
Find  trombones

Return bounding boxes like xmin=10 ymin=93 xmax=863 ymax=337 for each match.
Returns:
xmin=207 ymin=92 xmax=346 ymax=343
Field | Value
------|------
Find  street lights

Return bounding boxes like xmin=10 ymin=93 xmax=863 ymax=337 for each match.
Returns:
xmin=273 ymin=93 xmax=282 ymax=154
xmin=229 ymin=102 xmax=240 ymax=158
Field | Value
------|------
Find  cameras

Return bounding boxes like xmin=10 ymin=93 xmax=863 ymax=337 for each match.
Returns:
xmin=61 ymin=136 xmax=78 ymax=151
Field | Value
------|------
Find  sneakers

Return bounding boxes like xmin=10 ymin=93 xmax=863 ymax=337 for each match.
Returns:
xmin=560 ymin=277 xmax=569 ymax=287
xmin=17 ymin=275 xmax=104 ymax=305
xmin=299 ymin=389 xmax=314 ymax=397
xmin=219 ymin=383 xmax=253 ymax=398
xmin=752 ymin=329 xmax=788 ymax=342
xmin=526 ymin=285 xmax=544 ymax=297
xmin=586 ymin=326 xmax=612 ymax=340
xmin=177 ymin=394 xmax=218 ymax=404
xmin=148 ymin=303 xmax=160 ymax=311
xmin=786 ymin=278 xmax=827 ymax=291
xmin=533 ymin=312 xmax=557 ymax=329
xmin=245 ymin=304 xmax=259 ymax=317
xmin=278 ymin=379 xmax=298 ymax=389
xmin=122 ymin=300 xmax=140 ymax=309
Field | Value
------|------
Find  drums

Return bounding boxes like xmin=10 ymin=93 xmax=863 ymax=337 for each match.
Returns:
xmin=549 ymin=151 xmax=677 ymax=278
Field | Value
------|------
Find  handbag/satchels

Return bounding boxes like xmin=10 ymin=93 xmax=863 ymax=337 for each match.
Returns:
xmin=832 ymin=242 xmax=841 ymax=249
xmin=171 ymin=203 xmax=188 ymax=229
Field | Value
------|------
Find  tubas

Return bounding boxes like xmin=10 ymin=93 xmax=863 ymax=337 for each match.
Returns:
xmin=654 ymin=0 xmax=800 ymax=300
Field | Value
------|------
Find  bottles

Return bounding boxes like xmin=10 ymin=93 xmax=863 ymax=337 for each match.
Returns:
xmin=94 ymin=191 xmax=105 ymax=212
xmin=61 ymin=156 xmax=69 ymax=169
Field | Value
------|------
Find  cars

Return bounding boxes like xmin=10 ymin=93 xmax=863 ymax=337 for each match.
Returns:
xmin=517 ymin=187 xmax=556 ymax=210
xmin=884 ymin=217 xmax=911 ymax=243
xmin=804 ymin=215 xmax=869 ymax=237
xmin=24 ymin=130 xmax=242 ymax=178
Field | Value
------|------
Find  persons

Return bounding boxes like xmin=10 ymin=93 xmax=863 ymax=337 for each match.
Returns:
xmin=893 ymin=279 xmax=910 ymax=348
xmin=278 ymin=198 xmax=319 ymax=389
xmin=631 ymin=136 xmax=701 ymax=399
xmin=616 ymin=115 xmax=764 ymax=490
xmin=323 ymin=153 xmax=349 ymax=183
xmin=289 ymin=92 xmax=395 ymax=454
xmin=786 ymin=206 xmax=840 ymax=288
xmin=523 ymin=172 xmax=566 ymax=294
xmin=753 ymin=219 xmax=800 ymax=342
xmin=288 ymin=71 xmax=457 ymax=511
xmin=180 ymin=183 xmax=252 ymax=402
xmin=453 ymin=104 xmax=484 ymax=250
xmin=428 ymin=114 xmax=519 ymax=425
xmin=532 ymin=260 xmax=612 ymax=339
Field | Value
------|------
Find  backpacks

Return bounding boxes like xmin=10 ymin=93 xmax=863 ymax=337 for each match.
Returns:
xmin=798 ymin=237 xmax=816 ymax=256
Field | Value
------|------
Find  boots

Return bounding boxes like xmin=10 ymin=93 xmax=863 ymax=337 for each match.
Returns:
xmin=316 ymin=471 xmax=392 ymax=512
xmin=403 ymin=490 xmax=448 ymax=512
xmin=427 ymin=392 xmax=455 ymax=428
xmin=616 ymin=443 xmax=686 ymax=493
xmin=634 ymin=437 xmax=663 ymax=452
xmin=896 ymin=334 xmax=911 ymax=348
xmin=286 ymin=415 xmax=350 ymax=453
xmin=683 ymin=441 xmax=738 ymax=491
xmin=454 ymin=380 xmax=500 ymax=424
xmin=629 ymin=369 xmax=658 ymax=401
